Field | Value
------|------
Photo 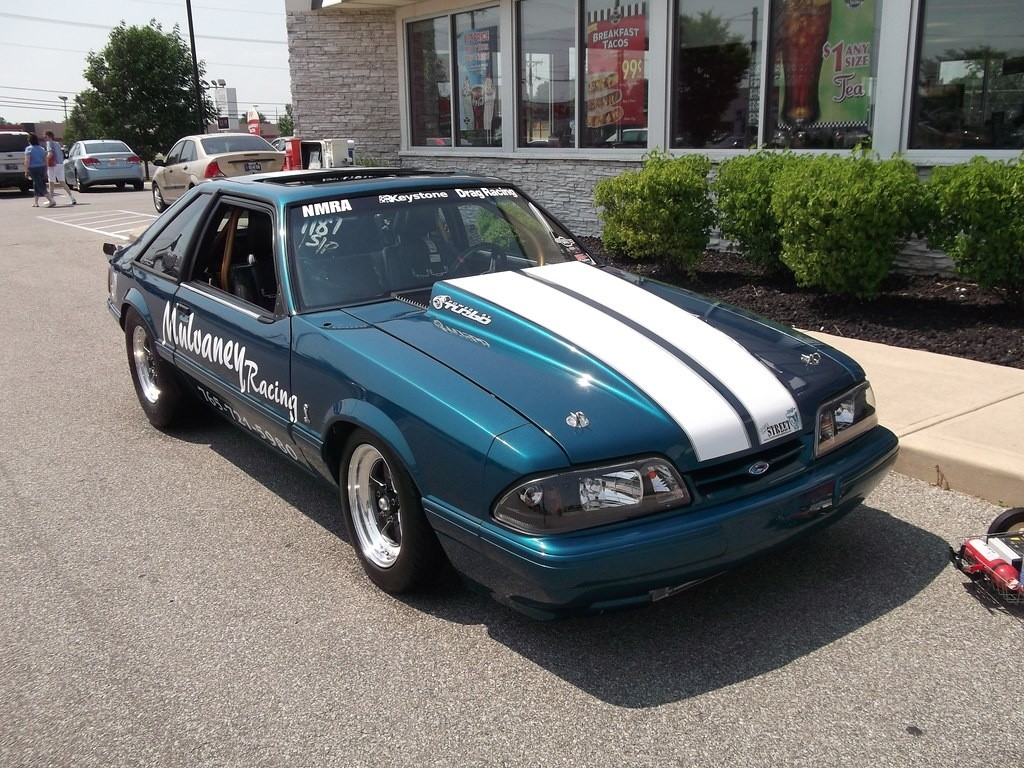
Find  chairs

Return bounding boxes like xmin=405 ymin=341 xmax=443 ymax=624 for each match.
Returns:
xmin=383 ymin=204 xmax=469 ymax=293
xmin=295 ymin=217 xmax=381 ymax=304
xmin=229 ymin=145 xmax=248 ymax=151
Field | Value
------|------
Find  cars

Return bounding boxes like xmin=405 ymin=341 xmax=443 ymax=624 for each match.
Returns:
xmin=64 ymin=139 xmax=144 ymax=193
xmin=151 ymin=132 xmax=293 ymax=213
xmin=103 ymin=166 xmax=901 ymax=622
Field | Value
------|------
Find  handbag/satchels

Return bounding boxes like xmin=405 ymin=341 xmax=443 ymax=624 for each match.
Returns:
xmin=48 ymin=156 xmax=57 ymax=166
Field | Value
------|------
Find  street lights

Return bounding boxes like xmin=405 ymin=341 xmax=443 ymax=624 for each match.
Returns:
xmin=58 ymin=95 xmax=69 ymax=129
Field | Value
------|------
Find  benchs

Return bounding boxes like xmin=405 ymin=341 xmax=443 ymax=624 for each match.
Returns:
xmin=232 ymin=230 xmax=387 ymax=307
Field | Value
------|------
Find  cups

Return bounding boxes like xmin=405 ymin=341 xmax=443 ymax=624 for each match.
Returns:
xmin=776 ymin=0 xmax=832 ymax=125
xmin=461 ymin=84 xmax=495 ymax=130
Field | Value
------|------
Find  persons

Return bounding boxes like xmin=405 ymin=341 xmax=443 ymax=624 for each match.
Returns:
xmin=42 ymin=131 xmax=76 ymax=206
xmin=25 ymin=134 xmax=56 ymax=208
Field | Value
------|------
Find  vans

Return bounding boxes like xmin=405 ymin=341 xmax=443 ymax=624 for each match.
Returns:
xmin=0 ymin=129 xmax=34 ymax=192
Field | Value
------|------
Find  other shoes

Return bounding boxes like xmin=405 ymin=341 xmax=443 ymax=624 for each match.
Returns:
xmin=48 ymin=201 xmax=56 ymax=207
xmin=44 ymin=201 xmax=50 ymax=205
xmin=71 ymin=199 xmax=76 ymax=204
xmin=32 ymin=204 xmax=39 ymax=207
